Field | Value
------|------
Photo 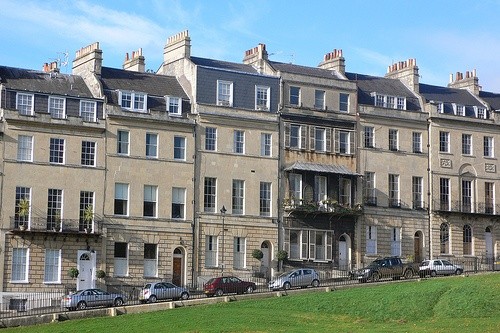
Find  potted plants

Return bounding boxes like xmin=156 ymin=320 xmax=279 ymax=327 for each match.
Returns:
xmin=52 ymin=210 xmax=62 ymax=232
xmin=68 ymin=267 xmax=80 ymax=295
xmin=15 ymin=198 xmax=30 ymax=230
xmin=84 ymin=206 xmax=95 ymax=233
xmin=251 ymin=248 xmax=264 ymax=276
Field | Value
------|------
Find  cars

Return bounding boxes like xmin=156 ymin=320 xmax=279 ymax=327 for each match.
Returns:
xmin=420 ymin=259 xmax=464 ymax=277
xmin=138 ymin=282 xmax=190 ymax=303
xmin=202 ymin=276 xmax=257 ymax=297
xmin=268 ymin=268 xmax=320 ymax=292
xmin=62 ymin=288 xmax=128 ymax=311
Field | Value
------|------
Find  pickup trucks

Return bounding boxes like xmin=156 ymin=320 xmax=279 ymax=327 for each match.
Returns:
xmin=349 ymin=256 xmax=420 ymax=284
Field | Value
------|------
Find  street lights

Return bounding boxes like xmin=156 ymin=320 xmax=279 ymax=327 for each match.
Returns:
xmin=220 ymin=205 xmax=228 ymax=276
